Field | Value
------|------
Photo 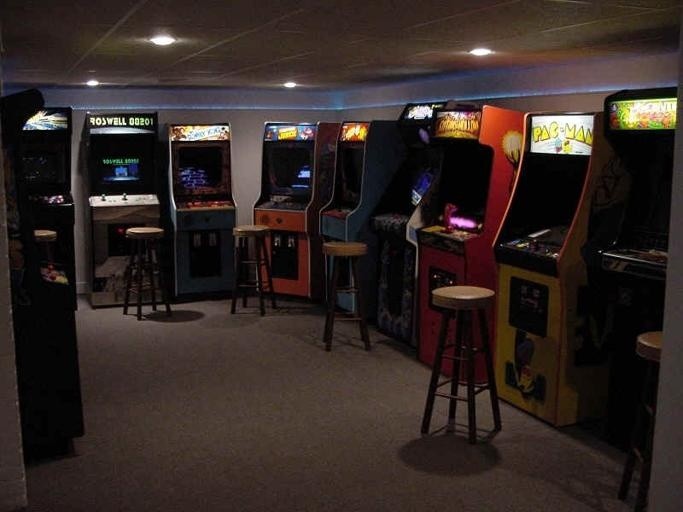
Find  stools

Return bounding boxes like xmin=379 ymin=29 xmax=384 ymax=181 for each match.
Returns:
xmin=615 ymin=330 xmax=664 ymax=511
xmin=320 ymin=241 xmax=373 ymax=351
xmin=119 ymin=225 xmax=173 ymax=321
xmin=417 ymin=284 xmax=502 ymax=446
xmin=228 ymin=223 xmax=276 ymax=317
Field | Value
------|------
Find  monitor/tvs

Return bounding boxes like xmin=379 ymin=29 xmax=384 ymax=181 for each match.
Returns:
xmin=341 ymin=148 xmax=364 ymax=203
xmin=456 ymin=167 xmax=491 ymax=220
xmin=628 ymin=143 xmax=674 ymax=234
xmin=272 ymin=147 xmax=311 ymax=188
xmin=399 ymin=163 xmax=439 ymax=214
xmin=536 ymin=173 xmax=586 ymax=231
xmin=174 ymin=144 xmax=227 ymax=193
xmin=98 ymin=151 xmax=146 ymax=186
